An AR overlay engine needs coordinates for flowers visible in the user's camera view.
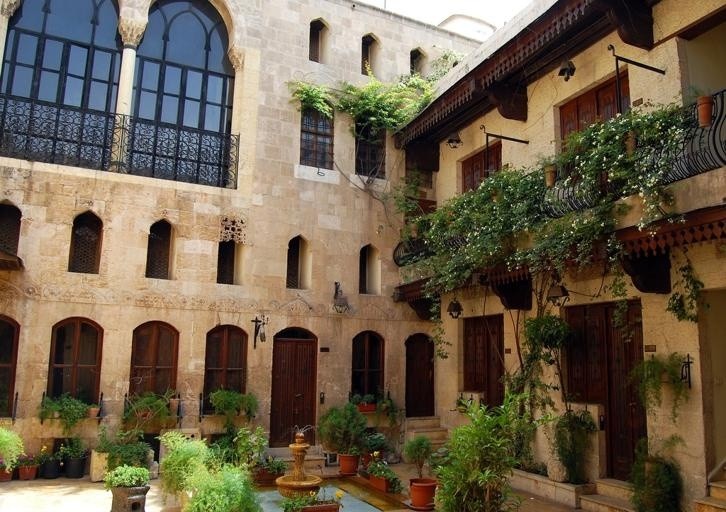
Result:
[276,489,344,512]
[380,87,710,358]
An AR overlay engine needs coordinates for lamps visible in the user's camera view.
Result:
[333,282,351,314]
[250,314,270,350]
[558,52,576,81]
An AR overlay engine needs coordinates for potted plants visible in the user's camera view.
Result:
[625,431,689,512]
[317,393,466,512]
[686,84,714,128]
[0,388,286,511]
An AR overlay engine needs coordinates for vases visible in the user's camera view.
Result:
[292,500,340,512]
